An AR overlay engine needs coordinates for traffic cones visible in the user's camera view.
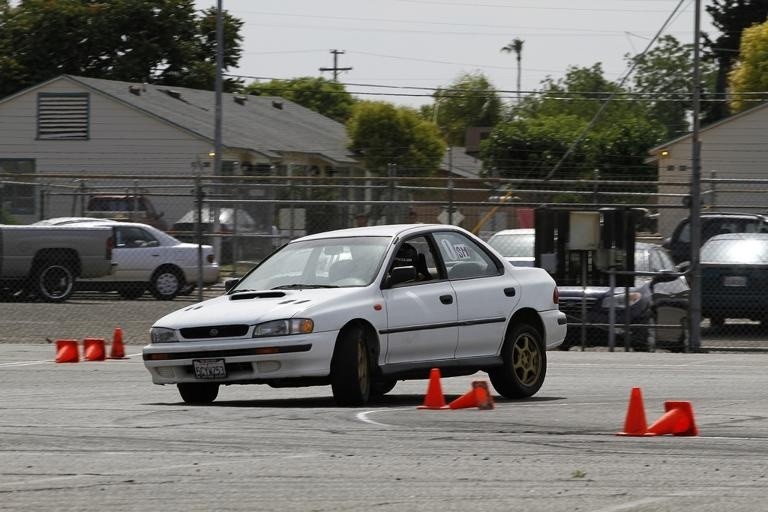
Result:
[614,386,701,441]
[51,322,129,366]
[420,361,497,413]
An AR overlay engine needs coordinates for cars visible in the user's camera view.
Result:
[0,192,261,300]
[487,213,768,350]
[141,220,571,402]
